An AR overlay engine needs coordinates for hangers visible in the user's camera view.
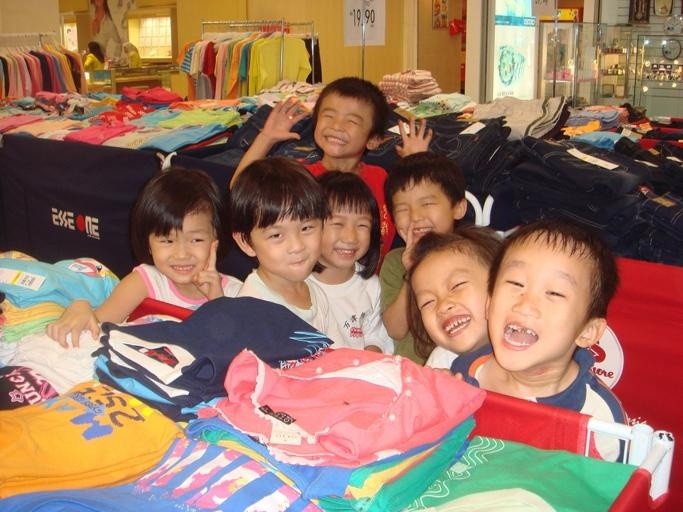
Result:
[184,20,318,47]
[229,21,315,85]
[200,19,285,82]
[0,32,81,64]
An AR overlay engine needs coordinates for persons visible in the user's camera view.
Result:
[306,171,395,355]
[230,158,348,350]
[229,77,433,276]
[379,151,468,367]
[429,216,630,465]
[81,41,104,83]
[45,166,244,350]
[402,226,502,371]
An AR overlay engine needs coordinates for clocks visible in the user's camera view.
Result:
[661,38,681,61]
[663,15,683,35]
[653,2,672,18]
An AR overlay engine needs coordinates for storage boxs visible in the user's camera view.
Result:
[126,297,681,512]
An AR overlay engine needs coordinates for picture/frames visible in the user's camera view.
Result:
[628,0,650,25]
